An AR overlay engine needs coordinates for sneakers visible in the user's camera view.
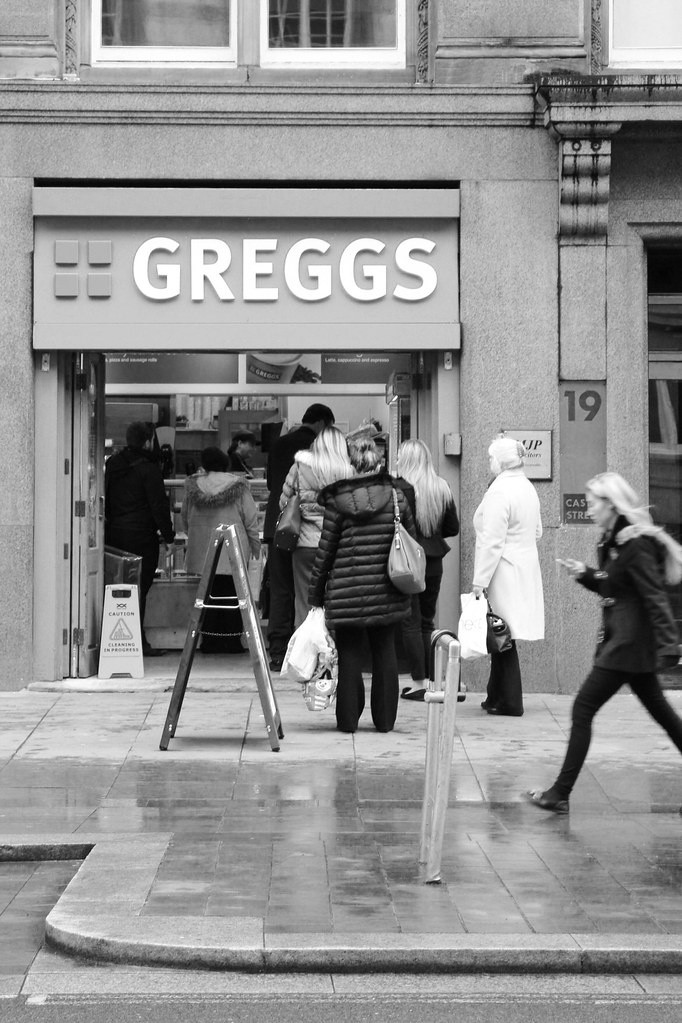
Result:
[267,659,283,671]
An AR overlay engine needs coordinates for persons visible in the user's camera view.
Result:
[224,426,258,481]
[104,418,176,658]
[306,433,418,736]
[180,445,264,655]
[471,434,549,719]
[277,426,354,640]
[516,470,681,814]
[259,399,341,675]
[385,436,466,701]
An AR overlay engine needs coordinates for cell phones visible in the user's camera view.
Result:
[556,559,574,569]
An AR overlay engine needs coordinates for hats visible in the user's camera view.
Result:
[234,430,262,445]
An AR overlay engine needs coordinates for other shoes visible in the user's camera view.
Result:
[340,727,357,732]
[376,724,395,732]
[486,707,503,714]
[481,701,495,710]
[400,686,427,701]
[522,789,570,815]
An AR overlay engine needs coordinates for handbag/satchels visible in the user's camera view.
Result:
[302,627,339,710]
[273,494,302,552]
[279,607,322,681]
[387,522,427,593]
[458,592,488,661]
[487,613,513,653]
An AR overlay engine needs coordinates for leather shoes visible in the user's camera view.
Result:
[141,643,166,657]
